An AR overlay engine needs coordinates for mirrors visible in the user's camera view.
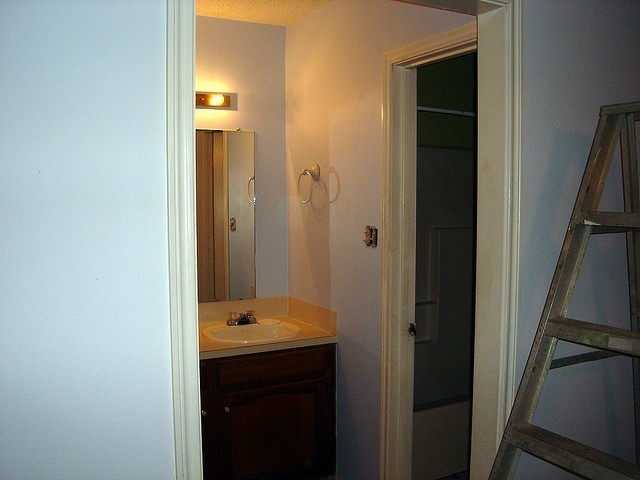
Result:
[197,130,257,304]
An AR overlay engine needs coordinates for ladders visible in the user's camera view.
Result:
[489,101,639,476]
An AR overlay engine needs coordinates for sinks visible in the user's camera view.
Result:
[202,318,303,343]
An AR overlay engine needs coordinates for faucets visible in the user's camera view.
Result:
[238,312,252,325]
